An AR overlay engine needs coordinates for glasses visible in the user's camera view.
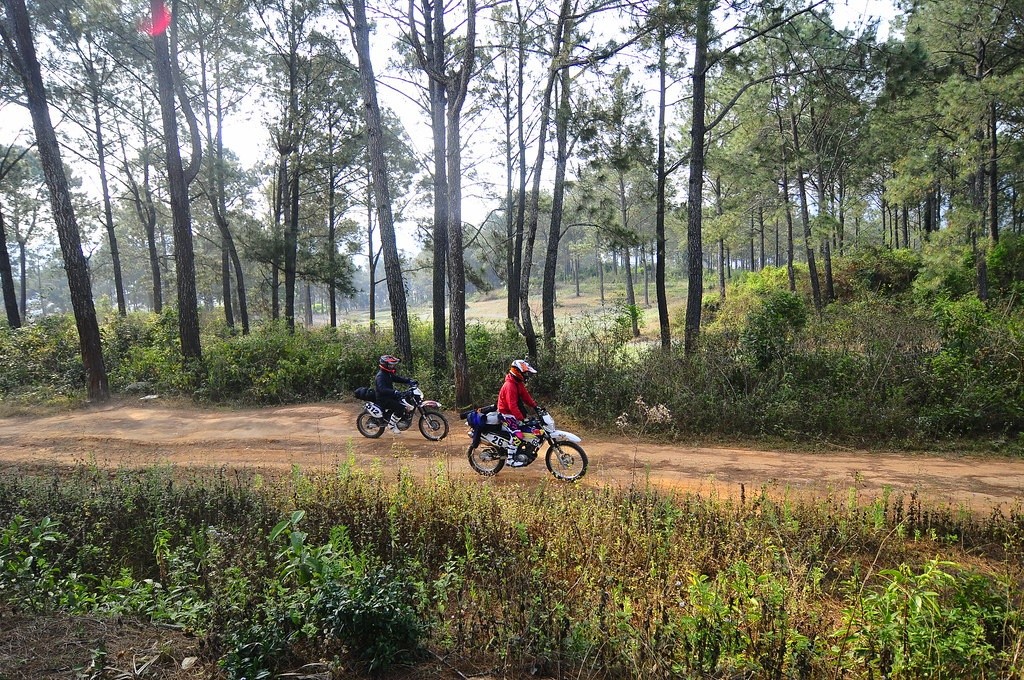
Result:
[523,372,532,377]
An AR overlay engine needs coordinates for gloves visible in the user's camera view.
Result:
[394,390,402,397]
[409,380,418,387]
[534,406,542,413]
[522,418,530,425]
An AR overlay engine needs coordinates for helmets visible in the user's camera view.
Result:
[379,355,400,374]
[509,360,537,382]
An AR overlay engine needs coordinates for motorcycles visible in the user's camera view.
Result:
[464,402,588,482]
[353,382,449,441]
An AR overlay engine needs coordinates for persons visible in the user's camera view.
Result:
[496,360,539,467]
[374,354,415,435]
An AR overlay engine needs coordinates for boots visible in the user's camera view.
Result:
[387,412,401,434]
[506,445,524,467]
